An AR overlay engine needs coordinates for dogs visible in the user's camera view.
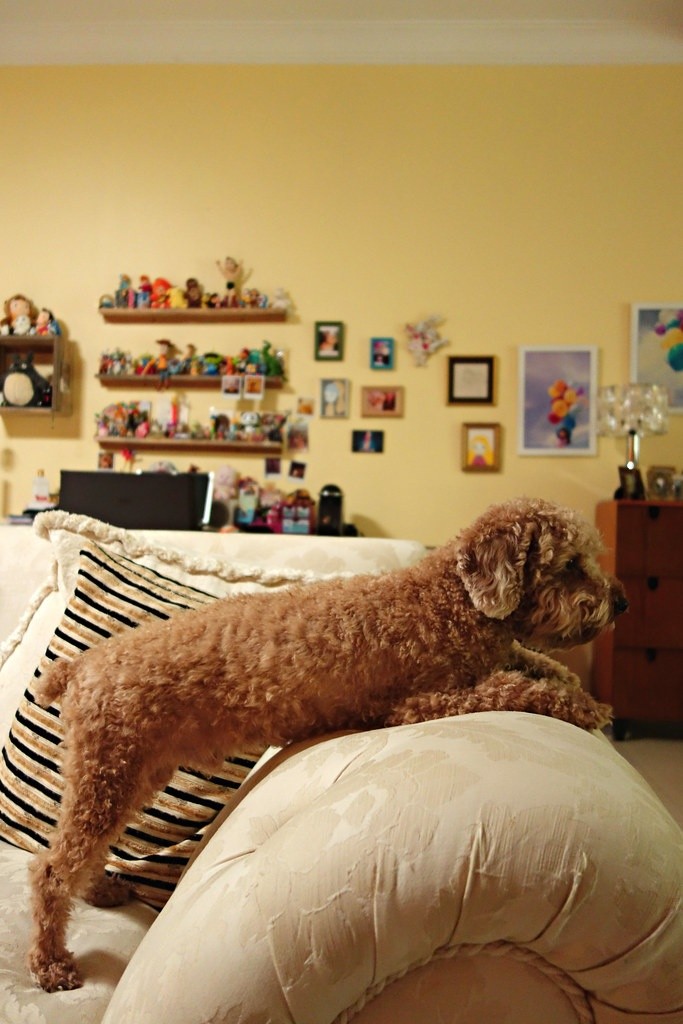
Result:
[30,495,628,984]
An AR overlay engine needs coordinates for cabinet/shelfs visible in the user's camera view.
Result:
[589,500,683,742]
[96,305,288,455]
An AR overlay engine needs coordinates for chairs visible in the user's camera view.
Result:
[22,469,217,534]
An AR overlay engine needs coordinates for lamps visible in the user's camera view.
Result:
[593,382,670,469]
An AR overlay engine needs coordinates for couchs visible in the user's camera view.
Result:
[1,521,683,1024]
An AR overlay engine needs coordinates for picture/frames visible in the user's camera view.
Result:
[628,300,682,416]
[448,355,494,405]
[516,345,599,457]
[314,320,344,362]
[461,422,501,473]
[320,377,349,419]
[369,336,395,371]
[361,386,405,418]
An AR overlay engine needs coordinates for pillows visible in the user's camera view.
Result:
[1,509,351,915]
